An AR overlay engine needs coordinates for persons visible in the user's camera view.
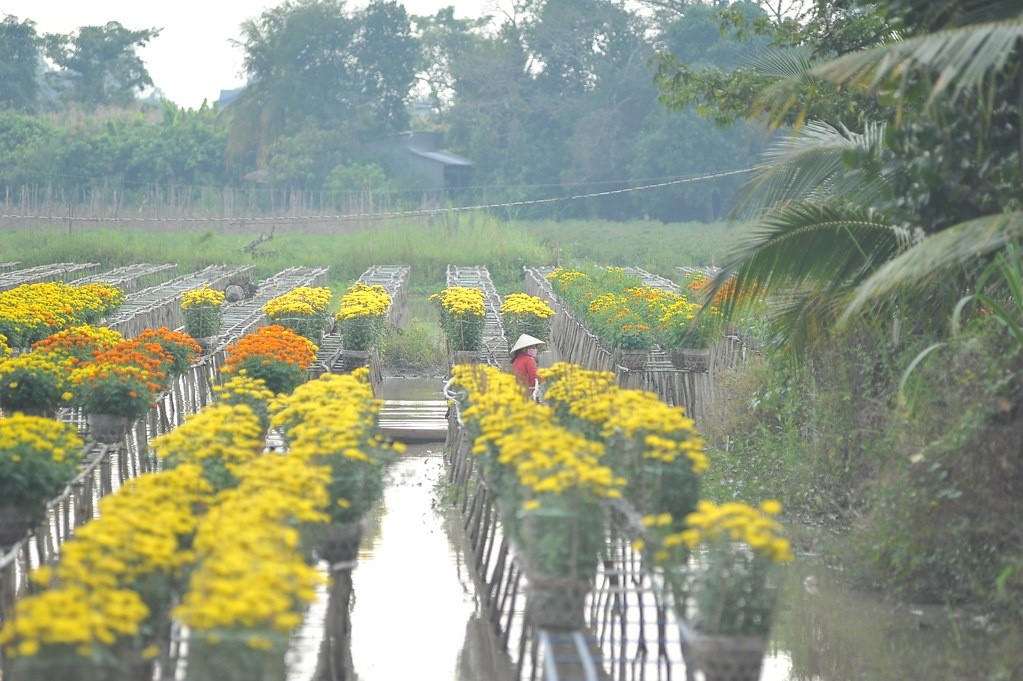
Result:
[509,334,546,400]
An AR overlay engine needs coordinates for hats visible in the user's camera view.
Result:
[510,334,546,354]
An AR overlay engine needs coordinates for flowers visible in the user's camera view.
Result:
[0,268,792,681]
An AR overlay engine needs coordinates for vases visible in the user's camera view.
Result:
[684,348,712,373]
[225,285,244,302]
[525,570,588,628]
[677,621,768,681]
[88,414,128,445]
[624,351,646,369]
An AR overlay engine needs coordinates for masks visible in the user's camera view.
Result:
[527,348,538,356]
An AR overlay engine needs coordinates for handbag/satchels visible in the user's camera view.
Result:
[528,378,545,403]
